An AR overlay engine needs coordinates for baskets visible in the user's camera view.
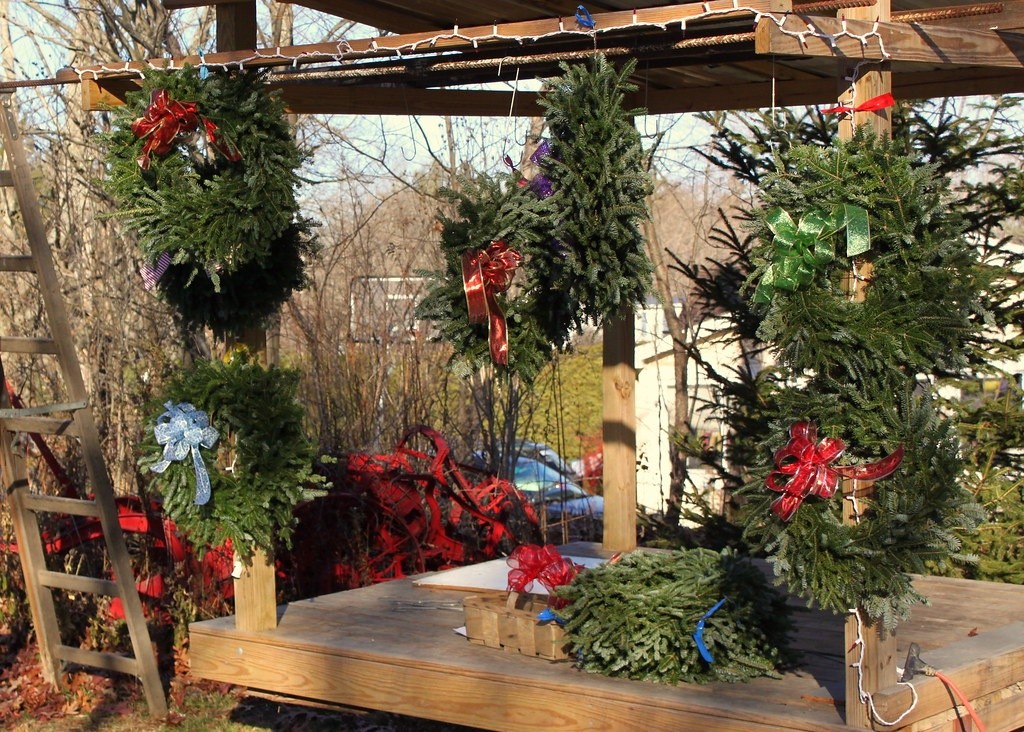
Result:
[462,554,576,660]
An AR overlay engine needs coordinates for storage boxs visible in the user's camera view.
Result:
[463,591,569,661]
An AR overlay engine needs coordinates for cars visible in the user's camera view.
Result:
[473,454,604,532]
[467,439,580,488]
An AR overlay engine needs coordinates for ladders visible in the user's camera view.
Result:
[0,88,170,722]
